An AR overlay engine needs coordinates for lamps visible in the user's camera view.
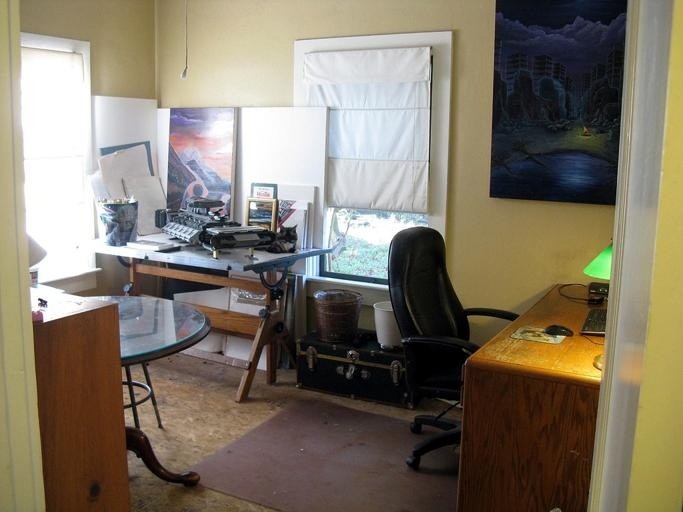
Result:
[581,237,614,281]
[26,233,48,268]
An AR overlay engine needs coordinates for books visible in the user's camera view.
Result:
[275,182,317,250]
[95,141,168,237]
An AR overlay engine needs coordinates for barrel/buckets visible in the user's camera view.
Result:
[314,289,363,343]
[97,199,138,246]
[373,301,402,346]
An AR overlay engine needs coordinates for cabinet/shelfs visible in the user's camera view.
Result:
[454,282,651,512]
[29,283,132,512]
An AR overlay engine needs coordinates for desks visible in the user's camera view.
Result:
[75,232,334,403]
[80,294,212,431]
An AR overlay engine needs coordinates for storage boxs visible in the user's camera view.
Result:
[295,328,425,411]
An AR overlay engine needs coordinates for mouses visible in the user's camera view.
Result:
[544,325,573,336]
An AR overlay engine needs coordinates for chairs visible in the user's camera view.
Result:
[387,227,521,470]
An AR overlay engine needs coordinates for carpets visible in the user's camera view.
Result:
[182,397,461,512]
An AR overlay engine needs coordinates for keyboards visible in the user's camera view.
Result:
[579,308,607,336]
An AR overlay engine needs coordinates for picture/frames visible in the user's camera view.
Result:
[245,182,278,232]
